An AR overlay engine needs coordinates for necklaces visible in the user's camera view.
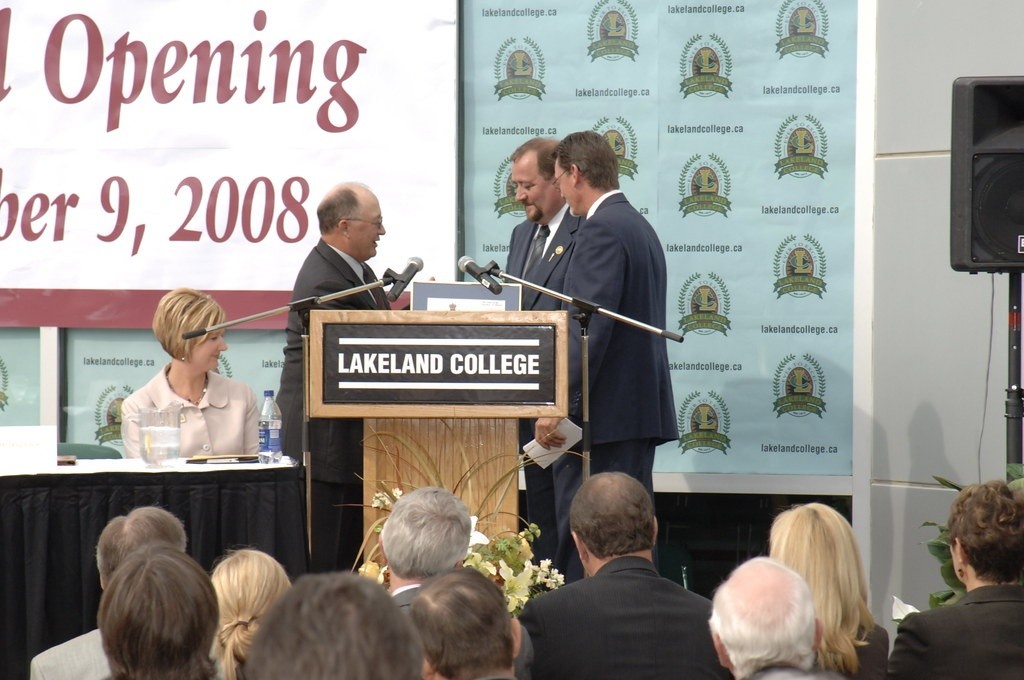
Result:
[166,373,208,405]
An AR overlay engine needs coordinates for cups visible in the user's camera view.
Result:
[136,408,182,465]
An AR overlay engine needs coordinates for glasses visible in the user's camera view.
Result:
[341,217,383,228]
[552,167,579,189]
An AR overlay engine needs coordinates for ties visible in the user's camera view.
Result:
[361,264,387,310]
[524,224,551,281]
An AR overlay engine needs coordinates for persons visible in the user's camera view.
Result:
[211,549,292,680]
[380,486,534,680]
[518,472,735,680]
[409,567,522,680]
[888,480,1024,680]
[275,183,392,571]
[29,506,186,680]
[243,572,423,680]
[709,556,824,680]
[507,139,585,567]
[536,130,679,590]
[120,289,260,459]
[769,503,890,680]
[97,541,221,680]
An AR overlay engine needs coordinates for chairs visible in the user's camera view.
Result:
[58,443,123,459]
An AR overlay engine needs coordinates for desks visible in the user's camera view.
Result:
[0,454,311,680]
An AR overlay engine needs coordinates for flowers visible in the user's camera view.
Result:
[331,419,593,616]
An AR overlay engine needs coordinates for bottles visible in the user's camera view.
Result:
[257,389,282,465]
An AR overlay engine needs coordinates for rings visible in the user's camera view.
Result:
[546,434,550,436]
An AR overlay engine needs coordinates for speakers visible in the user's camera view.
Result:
[951,75,1024,275]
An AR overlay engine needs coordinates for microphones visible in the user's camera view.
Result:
[386,257,424,302]
[457,256,502,295]
